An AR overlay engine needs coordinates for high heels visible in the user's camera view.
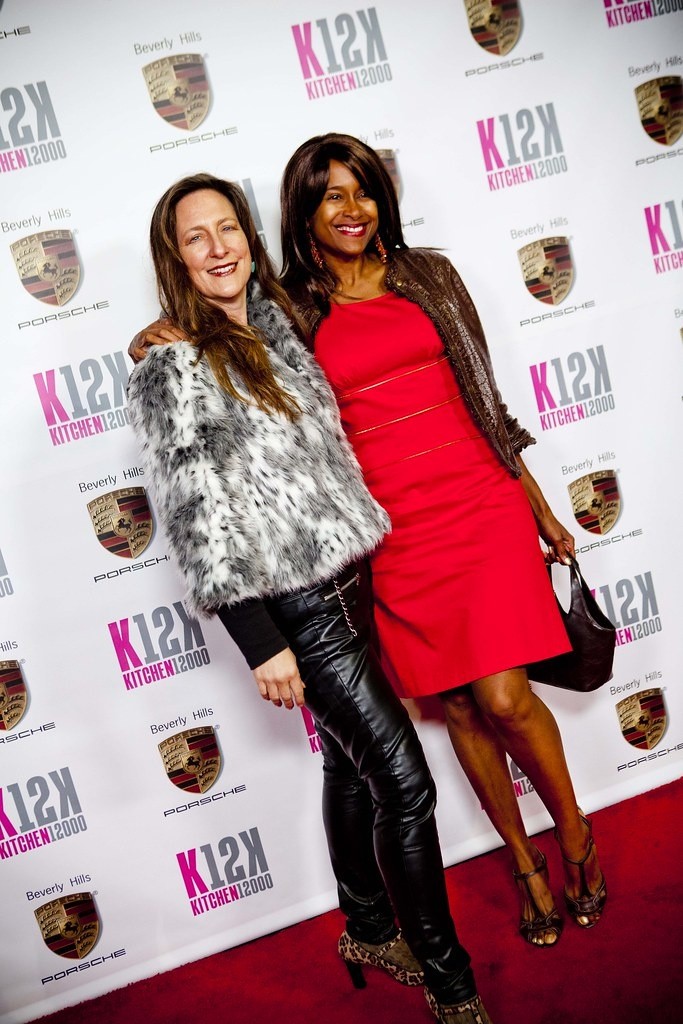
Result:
[554,814,606,928]
[512,845,563,945]
[337,927,424,990]
[424,985,491,1024]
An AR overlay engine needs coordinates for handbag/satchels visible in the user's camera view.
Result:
[526,551,617,692]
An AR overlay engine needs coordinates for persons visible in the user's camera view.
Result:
[127,133,608,947]
[125,172,490,1024]
